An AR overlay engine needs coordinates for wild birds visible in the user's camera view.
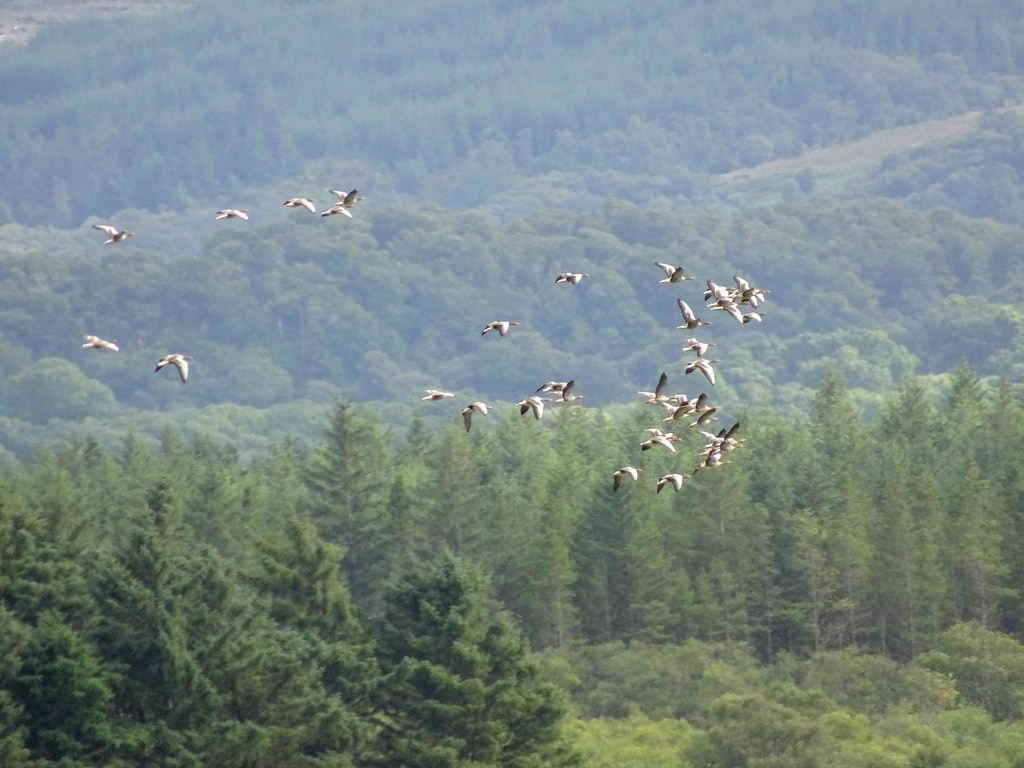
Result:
[214,208,249,222]
[535,381,567,394]
[329,190,366,207]
[636,272,771,475]
[652,260,695,285]
[320,204,353,219]
[481,321,519,336]
[555,380,584,404]
[462,401,493,434]
[422,389,455,401]
[283,197,317,213]
[610,466,642,491]
[154,352,193,382]
[81,334,121,353]
[515,395,552,421]
[555,272,589,284]
[655,472,691,494]
[91,224,133,246]
